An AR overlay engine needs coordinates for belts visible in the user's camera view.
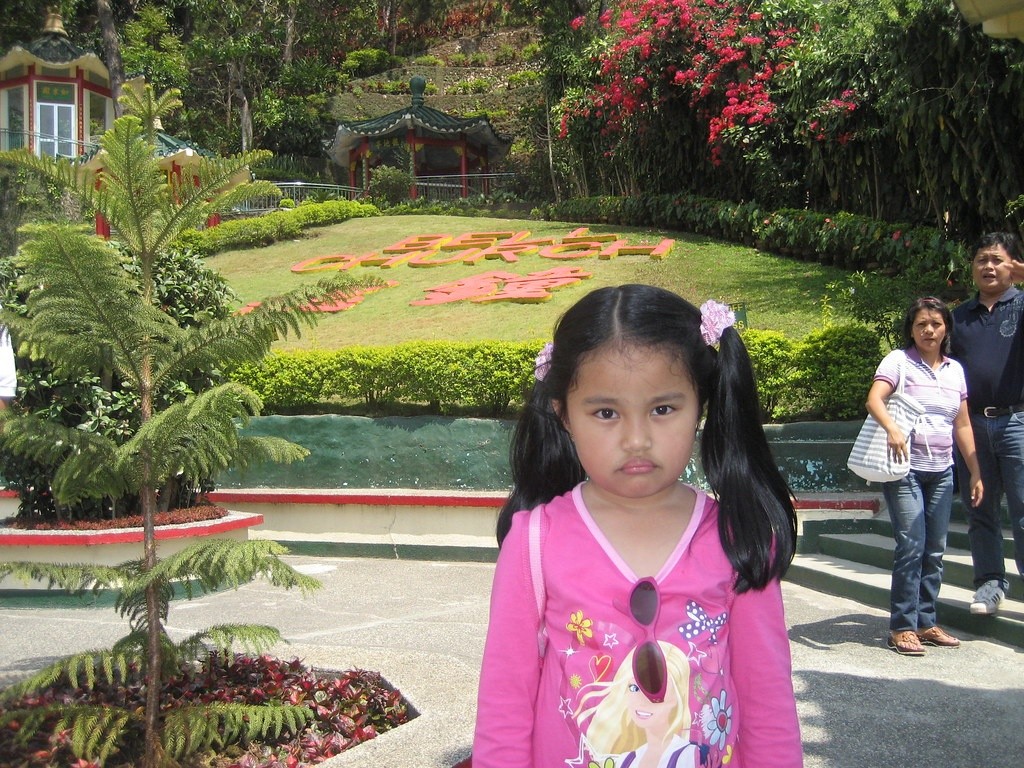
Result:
[969,403,1022,419]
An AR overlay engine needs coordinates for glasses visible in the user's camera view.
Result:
[630,577,668,703]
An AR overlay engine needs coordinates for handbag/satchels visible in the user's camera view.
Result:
[846,349,927,483]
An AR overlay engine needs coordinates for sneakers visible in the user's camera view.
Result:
[916,626,959,648]
[970,577,1009,615]
[887,625,927,655]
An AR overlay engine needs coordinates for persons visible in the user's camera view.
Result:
[471,285,804,768]
[942,232,1024,616]
[864,295,984,656]
